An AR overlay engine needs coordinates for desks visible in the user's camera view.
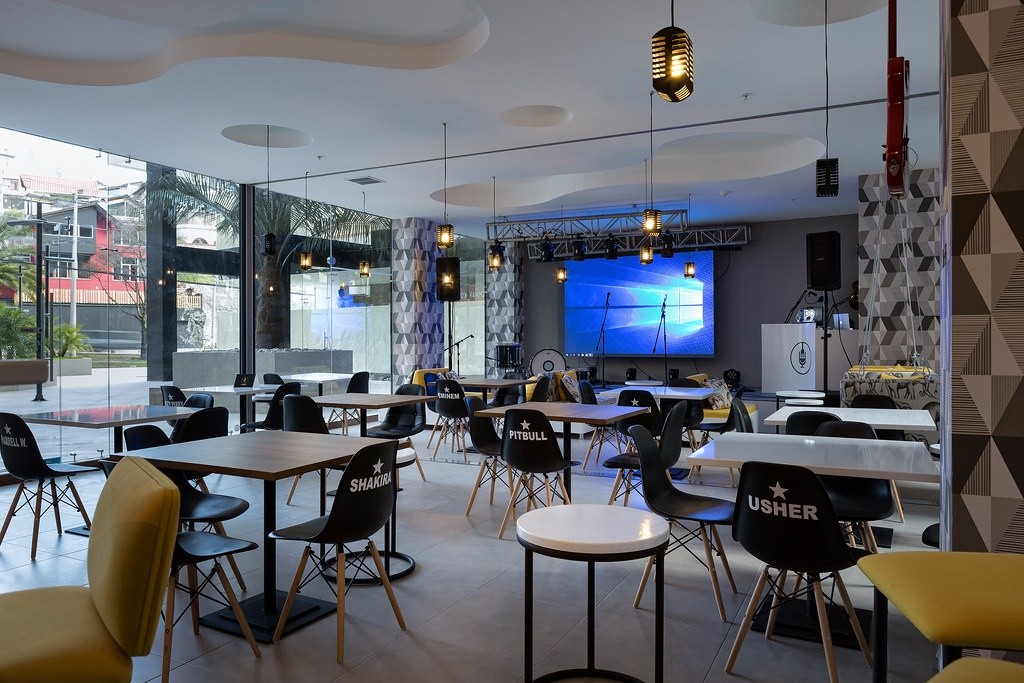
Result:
[21,372,941,682]
[761,323,859,393]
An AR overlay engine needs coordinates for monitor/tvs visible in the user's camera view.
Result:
[800,307,824,329]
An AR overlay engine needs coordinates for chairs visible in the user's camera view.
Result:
[0,373,895,683]
[855,550,1024,683]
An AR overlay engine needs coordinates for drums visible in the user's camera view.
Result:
[529,347,566,376]
[576,370,589,381]
[494,342,525,368]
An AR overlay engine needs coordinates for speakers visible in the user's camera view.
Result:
[436,256,460,302]
[805,231,841,291]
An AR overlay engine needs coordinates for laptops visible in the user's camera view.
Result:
[833,313,850,330]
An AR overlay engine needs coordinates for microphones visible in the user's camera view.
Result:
[605,292,610,305]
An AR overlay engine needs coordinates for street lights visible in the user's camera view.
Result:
[70,188,84,358]
[16,265,23,309]
[7,202,47,402]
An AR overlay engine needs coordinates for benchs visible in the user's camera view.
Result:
[378,369,618,440]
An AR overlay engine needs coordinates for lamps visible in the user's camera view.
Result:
[571,235,585,261]
[489,177,501,268]
[684,194,695,279]
[301,172,312,270]
[642,91,662,237]
[436,123,454,249]
[604,233,617,259]
[640,238,653,265]
[555,205,567,283]
[360,192,369,278]
[661,230,673,258]
[443,249,452,287]
[265,125,276,256]
[815,0,839,197]
[539,232,555,262]
[723,369,741,388]
[652,0,695,103]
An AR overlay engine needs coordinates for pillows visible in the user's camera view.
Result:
[436,372,446,380]
[525,375,538,401]
[561,373,581,403]
[444,370,464,392]
[556,369,578,401]
[403,371,413,384]
